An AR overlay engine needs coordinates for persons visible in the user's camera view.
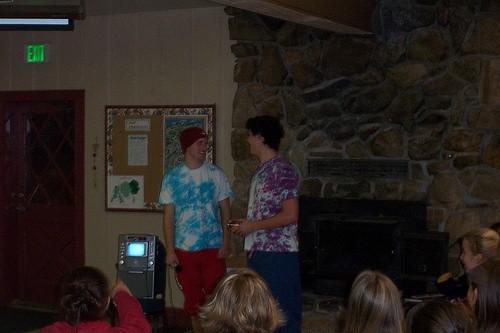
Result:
[159,127,234,318]
[190,269,283,333]
[33,266,152,333]
[339,221,500,333]
[227,114,302,333]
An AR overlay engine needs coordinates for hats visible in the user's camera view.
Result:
[179,127,208,154]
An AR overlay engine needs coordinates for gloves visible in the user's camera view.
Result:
[437,272,452,284]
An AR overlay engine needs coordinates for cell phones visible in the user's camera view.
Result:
[227,224,240,227]
